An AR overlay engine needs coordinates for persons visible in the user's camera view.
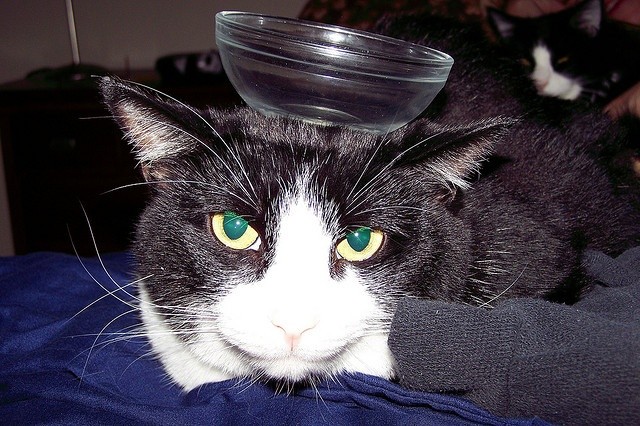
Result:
[603,83,640,177]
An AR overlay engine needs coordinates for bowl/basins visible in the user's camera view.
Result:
[214,11,454,135]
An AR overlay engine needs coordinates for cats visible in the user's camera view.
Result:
[98,2,640,395]
[483,0,640,118]
